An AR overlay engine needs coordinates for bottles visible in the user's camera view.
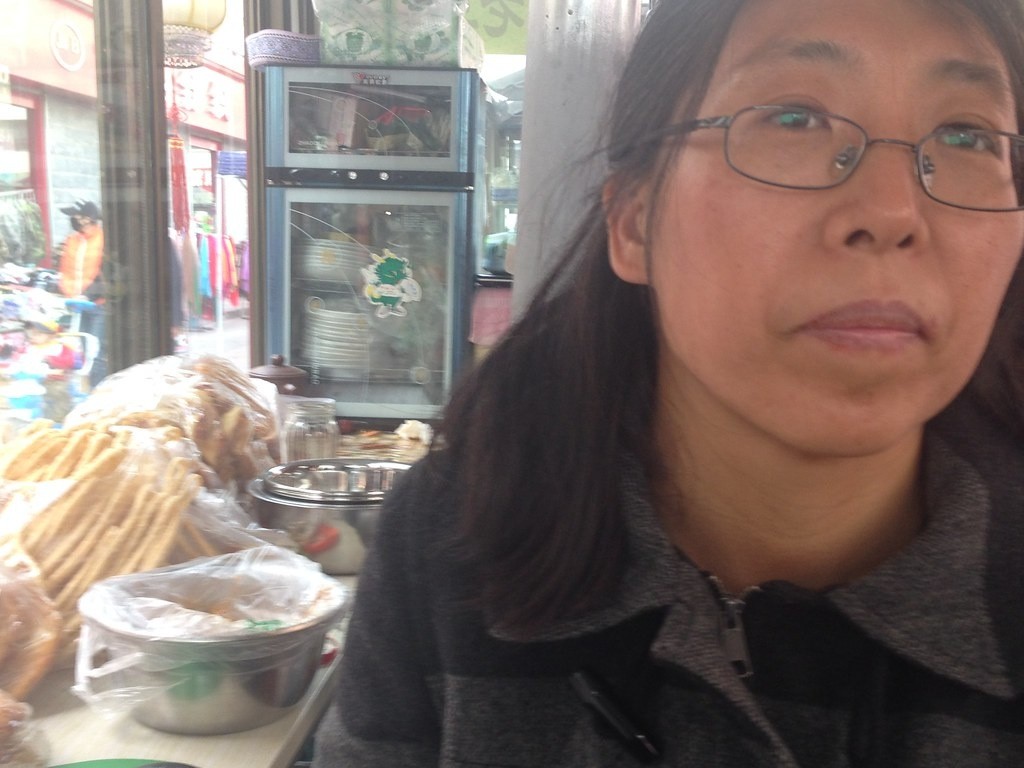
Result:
[280,396,339,461]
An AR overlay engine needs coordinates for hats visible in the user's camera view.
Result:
[60,198,98,219]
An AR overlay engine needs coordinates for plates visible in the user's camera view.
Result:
[290,237,377,379]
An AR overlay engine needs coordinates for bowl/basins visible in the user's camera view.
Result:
[76,575,348,736]
[245,456,412,577]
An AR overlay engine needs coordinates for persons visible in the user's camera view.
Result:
[310,1,1024,766]
[79,236,182,355]
[55,198,107,389]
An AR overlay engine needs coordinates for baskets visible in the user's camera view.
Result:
[247,29,323,71]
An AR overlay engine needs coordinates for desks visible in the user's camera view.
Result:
[26,655,344,768]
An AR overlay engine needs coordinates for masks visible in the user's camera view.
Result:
[70,217,93,231]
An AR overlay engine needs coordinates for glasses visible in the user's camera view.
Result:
[658,104,1024,212]
